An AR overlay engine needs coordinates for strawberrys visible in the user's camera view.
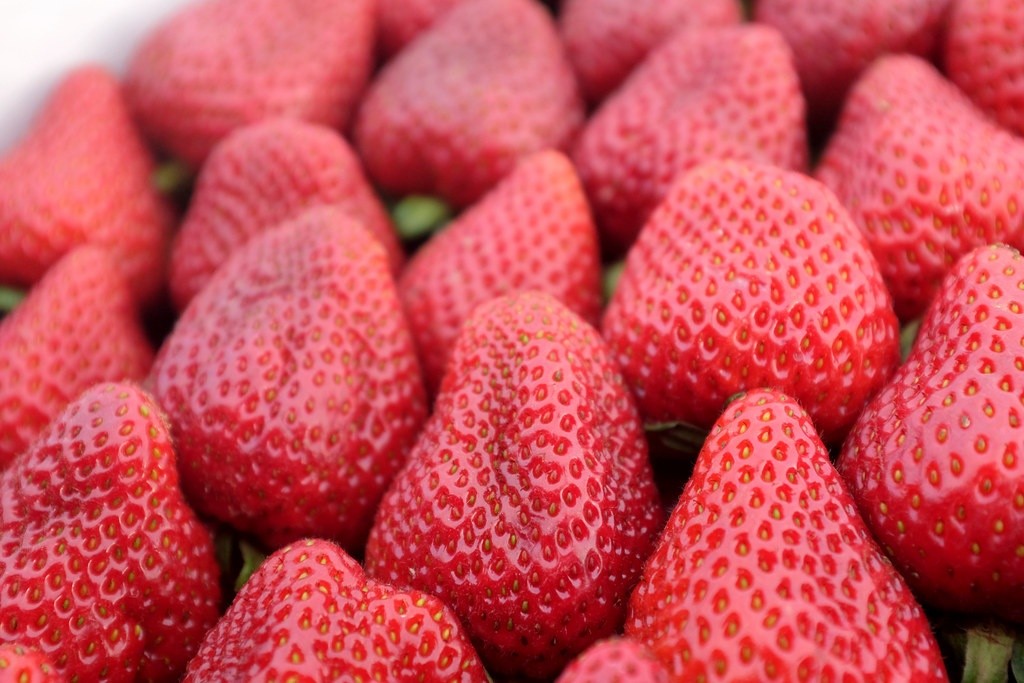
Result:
[0,0,1024,683]
